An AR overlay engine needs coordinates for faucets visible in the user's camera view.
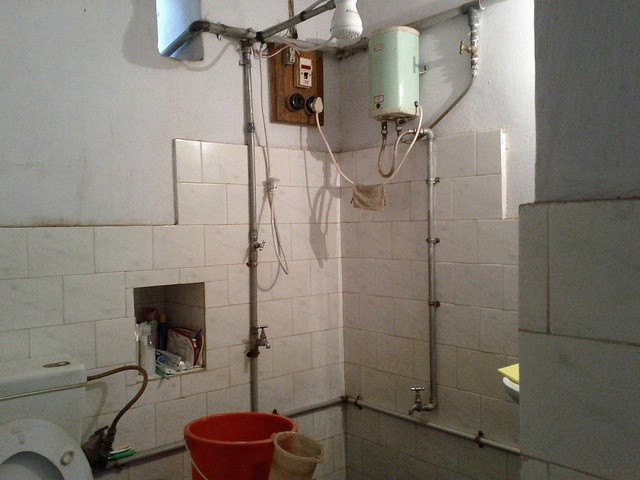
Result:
[255,325,271,350]
[408,387,424,414]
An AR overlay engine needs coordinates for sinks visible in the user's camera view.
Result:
[0,356,97,480]
[501,375,520,405]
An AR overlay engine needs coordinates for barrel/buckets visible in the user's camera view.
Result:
[183,412,298,480]
